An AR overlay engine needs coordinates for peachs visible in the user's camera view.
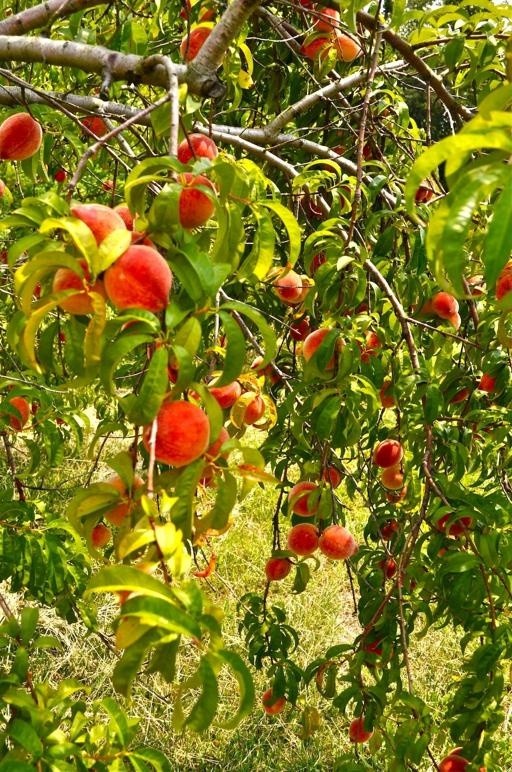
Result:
[1,1,511,772]
